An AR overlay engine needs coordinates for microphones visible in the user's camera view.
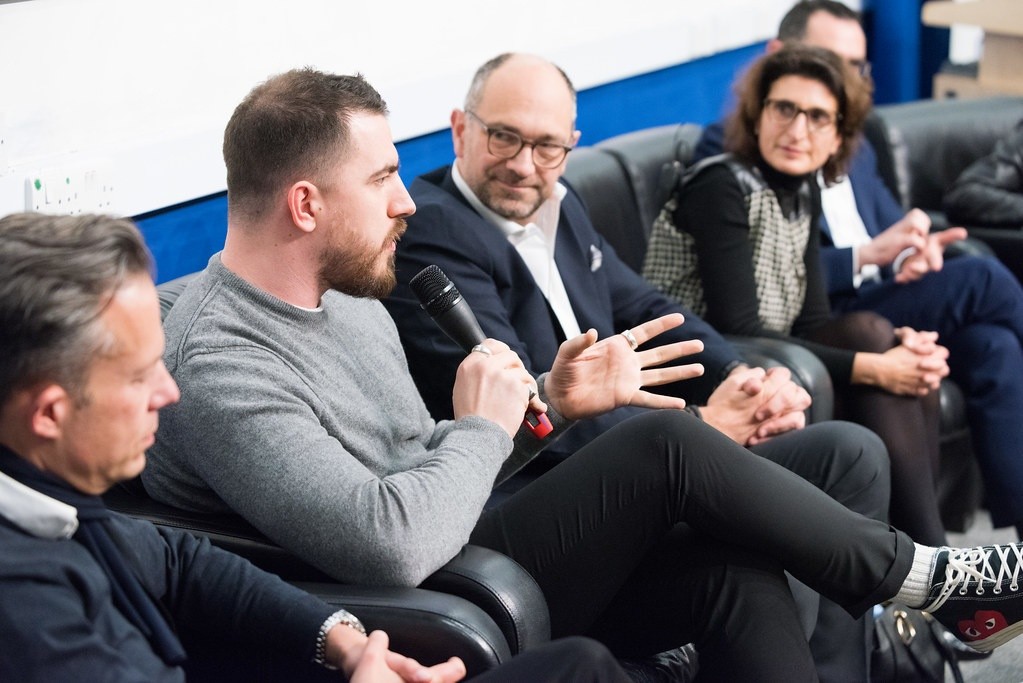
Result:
[409,264,554,439]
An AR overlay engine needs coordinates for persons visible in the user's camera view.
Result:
[379,52,891,682]
[638,38,996,659]
[141,68,1023,682]
[313,608,367,670]
[767,0,1023,541]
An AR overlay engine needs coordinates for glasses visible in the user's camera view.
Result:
[466,109,573,171]
[763,97,844,131]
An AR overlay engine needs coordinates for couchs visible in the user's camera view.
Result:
[0,91,1023,683]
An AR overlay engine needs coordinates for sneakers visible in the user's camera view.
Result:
[903,541,1023,652]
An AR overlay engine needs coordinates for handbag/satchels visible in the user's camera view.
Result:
[870,601,963,683]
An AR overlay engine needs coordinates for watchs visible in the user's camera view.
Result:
[0,213,633,683]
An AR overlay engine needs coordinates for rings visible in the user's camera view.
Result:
[621,329,638,350]
